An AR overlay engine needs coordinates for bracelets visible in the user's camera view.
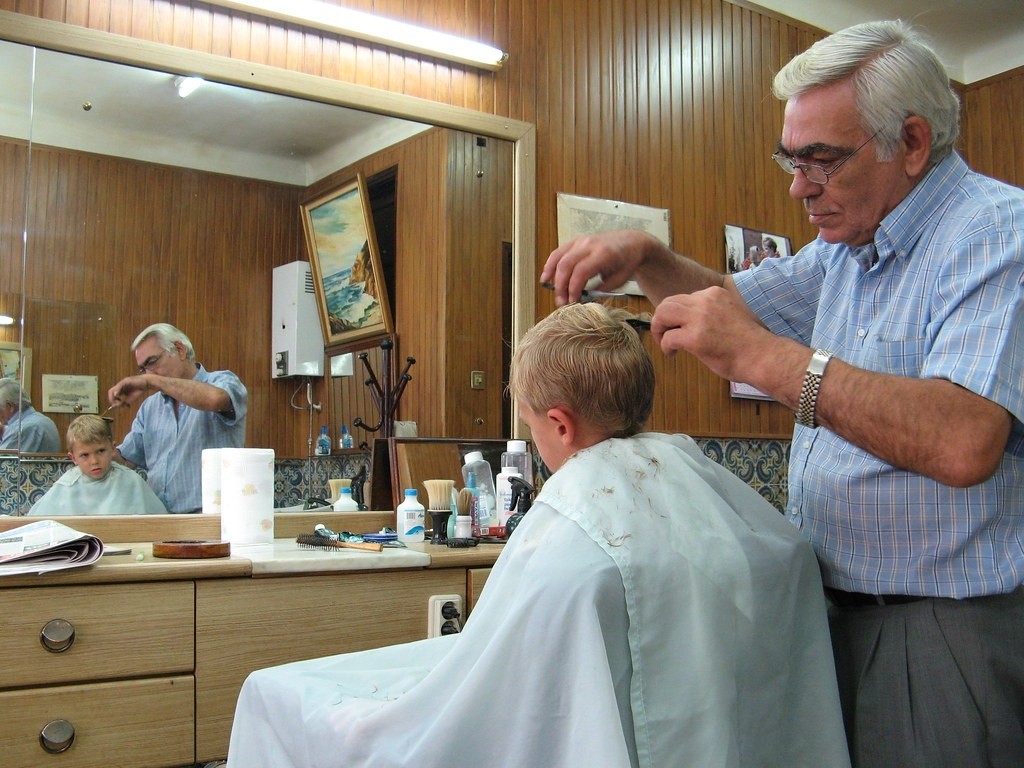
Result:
[792,348,830,428]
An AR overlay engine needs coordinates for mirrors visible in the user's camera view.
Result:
[0,9,538,543]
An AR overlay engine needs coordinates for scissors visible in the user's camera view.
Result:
[539,278,632,302]
[102,392,131,416]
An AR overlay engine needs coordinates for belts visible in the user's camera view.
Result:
[823,584,930,608]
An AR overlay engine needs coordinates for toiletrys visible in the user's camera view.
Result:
[315,424,331,455]
[339,423,353,449]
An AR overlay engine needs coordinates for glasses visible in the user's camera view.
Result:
[137,349,167,373]
[771,124,886,185]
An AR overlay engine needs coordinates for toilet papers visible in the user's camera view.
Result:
[221,448,275,541]
[201,447,220,515]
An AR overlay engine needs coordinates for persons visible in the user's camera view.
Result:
[109,323,248,515]
[540,20,1023,767]
[226,302,851,768]
[27,415,168,515]
[741,237,781,269]
[0,378,61,452]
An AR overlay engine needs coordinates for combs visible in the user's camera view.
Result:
[628,317,652,330]
[297,535,383,552]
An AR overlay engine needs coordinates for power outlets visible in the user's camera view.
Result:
[427,594,462,636]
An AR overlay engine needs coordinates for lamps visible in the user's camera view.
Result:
[197,0,509,72]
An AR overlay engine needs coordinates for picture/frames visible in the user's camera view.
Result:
[298,170,394,357]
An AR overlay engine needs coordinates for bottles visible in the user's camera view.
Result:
[338,424,352,447]
[396,489,425,543]
[461,472,481,537]
[455,515,472,538]
[332,488,358,513]
[497,467,524,528]
[501,441,535,506]
[314,426,330,456]
[460,450,500,536]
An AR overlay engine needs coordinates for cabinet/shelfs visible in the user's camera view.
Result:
[1,530,507,768]
